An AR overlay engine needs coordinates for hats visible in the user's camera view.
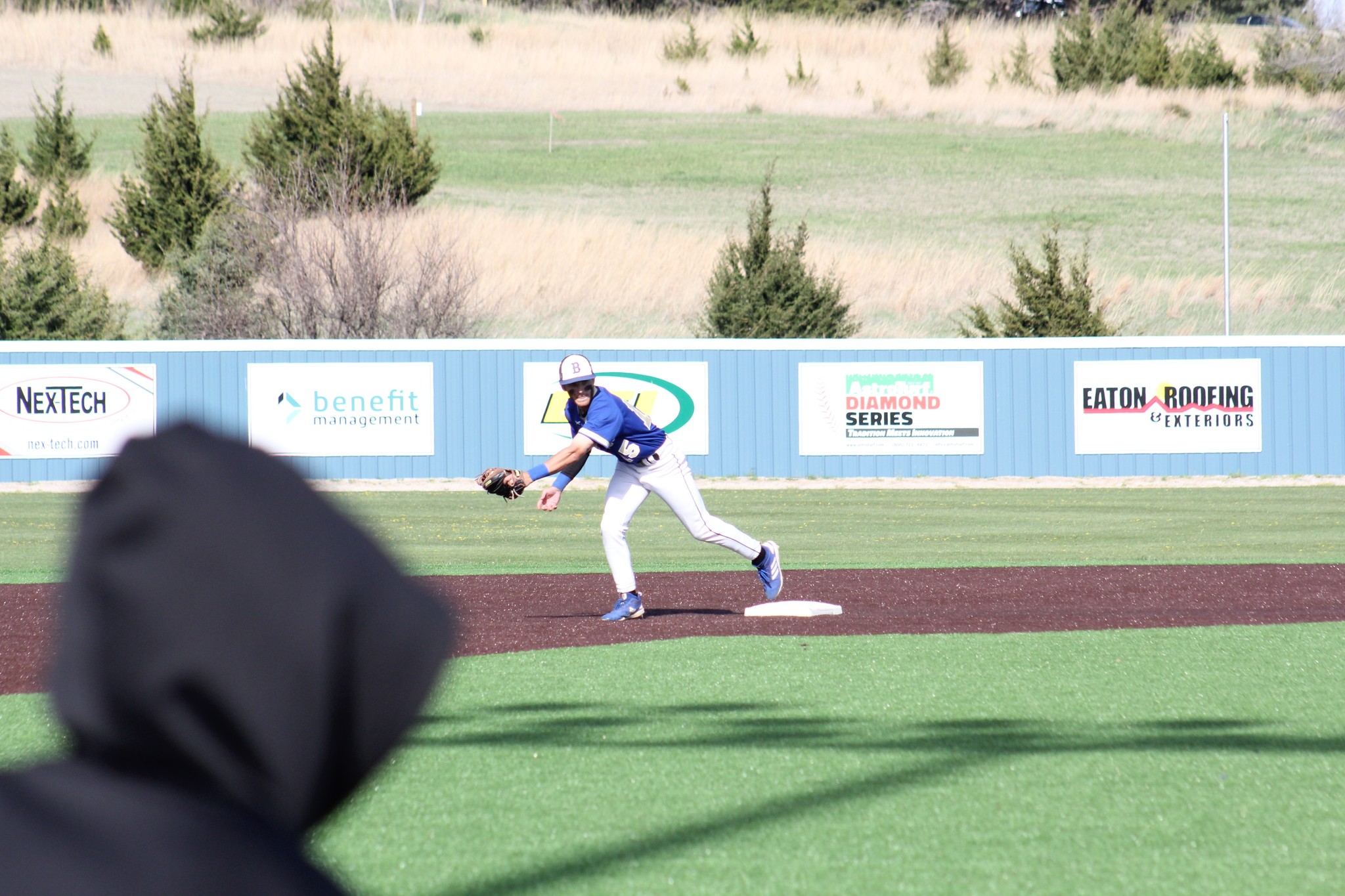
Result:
[559,354,596,385]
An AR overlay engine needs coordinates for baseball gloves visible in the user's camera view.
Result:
[476,468,526,500]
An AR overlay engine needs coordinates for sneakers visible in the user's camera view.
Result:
[751,540,783,600]
[601,593,645,621]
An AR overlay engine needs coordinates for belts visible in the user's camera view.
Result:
[634,453,660,467]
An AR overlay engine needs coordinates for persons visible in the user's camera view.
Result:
[502,354,783,620]
[0,425,455,896]
